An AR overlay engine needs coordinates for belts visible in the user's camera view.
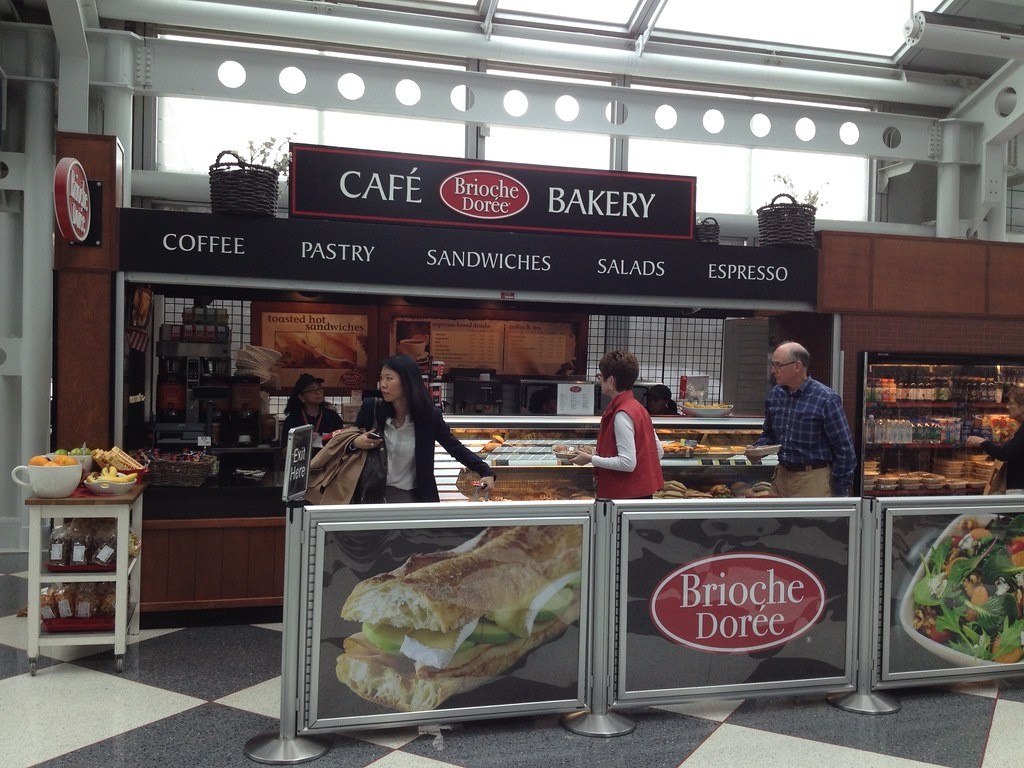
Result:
[779,463,831,472]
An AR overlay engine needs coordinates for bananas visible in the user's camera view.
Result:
[87,466,138,486]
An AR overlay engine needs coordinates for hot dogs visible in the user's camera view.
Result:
[336,524,583,711]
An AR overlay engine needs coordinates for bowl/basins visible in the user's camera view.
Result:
[677,402,734,417]
[745,443,781,456]
[399,338,425,357]
[864,452,994,489]
[234,344,283,384]
[117,468,146,484]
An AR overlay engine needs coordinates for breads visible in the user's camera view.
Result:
[684,402,729,408]
[653,480,771,499]
[485,435,505,451]
[663,442,709,452]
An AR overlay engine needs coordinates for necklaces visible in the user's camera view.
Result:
[395,420,401,427]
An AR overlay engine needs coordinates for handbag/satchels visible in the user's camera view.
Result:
[352,397,387,499]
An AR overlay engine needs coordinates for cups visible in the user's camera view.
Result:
[11,464,83,498]
[39,453,93,480]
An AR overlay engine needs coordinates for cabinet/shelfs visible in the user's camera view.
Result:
[854,350,1024,496]
[24,484,149,676]
[434,414,781,501]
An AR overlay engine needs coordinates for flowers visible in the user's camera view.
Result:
[773,172,830,208]
[696,217,702,225]
[247,132,297,196]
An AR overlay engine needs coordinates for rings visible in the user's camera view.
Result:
[371,442,374,447]
[374,444,377,447]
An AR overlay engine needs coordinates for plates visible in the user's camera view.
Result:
[83,473,138,495]
[898,514,1024,668]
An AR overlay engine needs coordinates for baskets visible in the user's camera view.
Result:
[147,453,217,487]
[756,194,817,247]
[696,217,721,245]
[209,150,280,217]
[119,468,146,484]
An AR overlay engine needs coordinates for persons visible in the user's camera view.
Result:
[742,341,858,497]
[280,373,344,459]
[528,387,557,415]
[322,353,497,504]
[644,384,681,415]
[567,348,664,499]
[966,384,1024,495]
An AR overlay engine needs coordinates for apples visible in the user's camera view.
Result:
[55,447,91,455]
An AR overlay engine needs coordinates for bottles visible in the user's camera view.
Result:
[211,404,221,417]
[240,403,252,418]
[162,403,178,417]
[866,376,1017,445]
[211,423,220,444]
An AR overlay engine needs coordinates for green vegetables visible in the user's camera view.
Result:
[915,512,1024,659]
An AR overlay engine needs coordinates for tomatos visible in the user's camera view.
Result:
[949,536,962,545]
[1011,541,1024,553]
[931,624,952,643]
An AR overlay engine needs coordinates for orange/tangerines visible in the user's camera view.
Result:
[29,455,78,467]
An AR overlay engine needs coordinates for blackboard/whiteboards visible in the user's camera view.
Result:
[281,424,313,502]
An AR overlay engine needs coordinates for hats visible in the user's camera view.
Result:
[296,373,325,384]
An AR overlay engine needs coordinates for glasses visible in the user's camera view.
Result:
[301,386,326,395]
[769,360,798,370]
[596,372,609,380]
